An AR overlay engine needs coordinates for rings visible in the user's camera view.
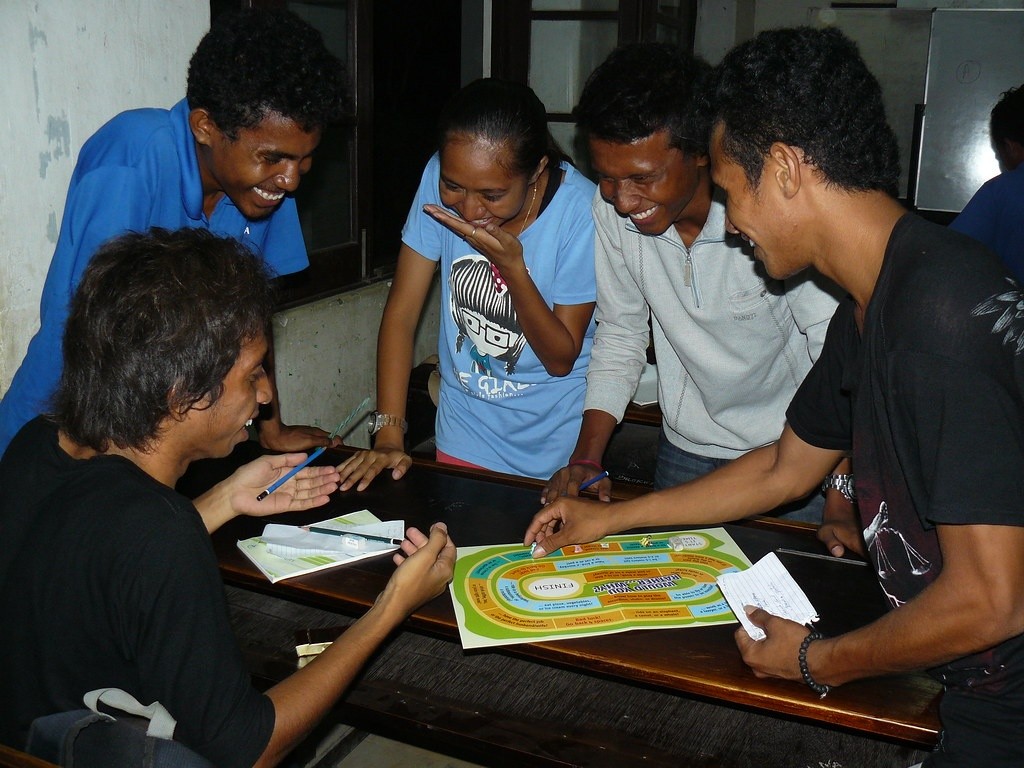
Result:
[463,234,466,240]
[472,227,477,237]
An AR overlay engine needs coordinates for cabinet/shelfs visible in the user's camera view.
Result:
[490,0,703,123]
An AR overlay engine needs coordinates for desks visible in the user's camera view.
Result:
[403,360,663,463]
[175,440,944,768]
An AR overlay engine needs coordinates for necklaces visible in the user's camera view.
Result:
[518,181,537,233]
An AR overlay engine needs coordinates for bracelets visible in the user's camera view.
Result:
[570,459,603,470]
[798,631,833,693]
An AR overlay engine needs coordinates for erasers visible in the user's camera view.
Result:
[342,534,366,548]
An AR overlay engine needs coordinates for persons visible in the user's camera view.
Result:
[524,26,1024,768]
[1,0,344,462]
[0,227,457,768]
[950,85,1024,283]
[540,45,869,681]
[334,78,597,492]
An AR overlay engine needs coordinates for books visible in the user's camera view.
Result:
[237,510,406,584]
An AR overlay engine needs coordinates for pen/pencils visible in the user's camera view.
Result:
[256,445,329,502]
[560,470,610,506]
[297,525,403,545]
[308,397,370,456]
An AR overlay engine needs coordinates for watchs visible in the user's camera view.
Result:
[821,474,857,506]
[368,410,408,434]
